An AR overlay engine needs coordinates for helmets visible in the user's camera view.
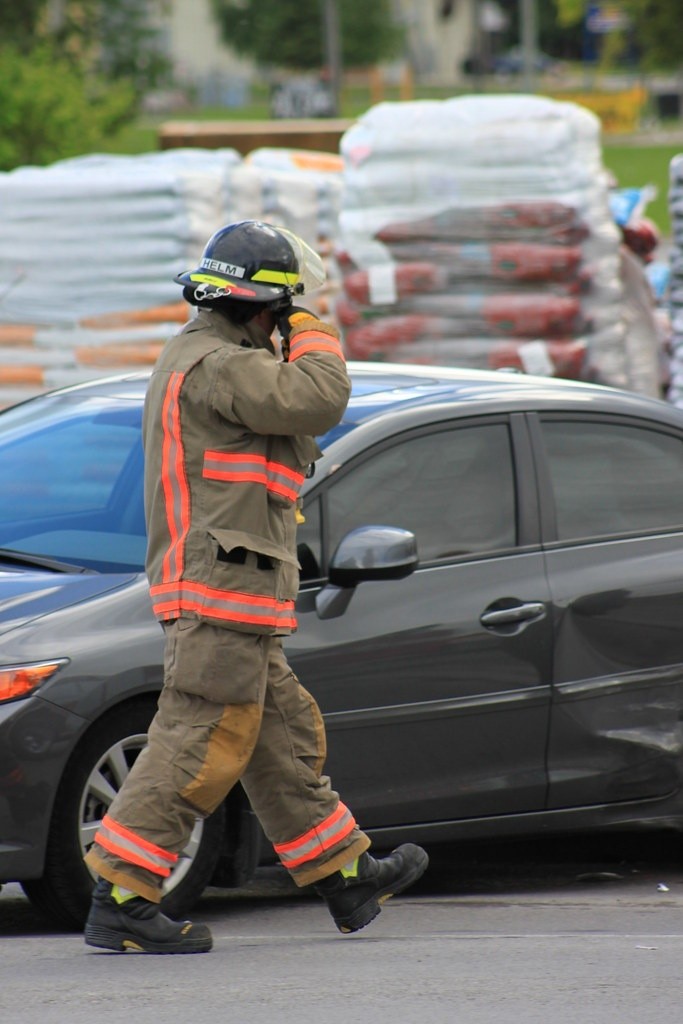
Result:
[173,220,305,301]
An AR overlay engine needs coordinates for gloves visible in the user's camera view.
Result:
[276,305,321,363]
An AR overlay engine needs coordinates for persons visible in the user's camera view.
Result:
[618,221,670,308]
[83,219,432,956]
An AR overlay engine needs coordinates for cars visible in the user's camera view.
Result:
[0,360,683,927]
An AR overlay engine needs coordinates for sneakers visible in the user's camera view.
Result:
[314,843,429,934]
[84,881,213,952]
